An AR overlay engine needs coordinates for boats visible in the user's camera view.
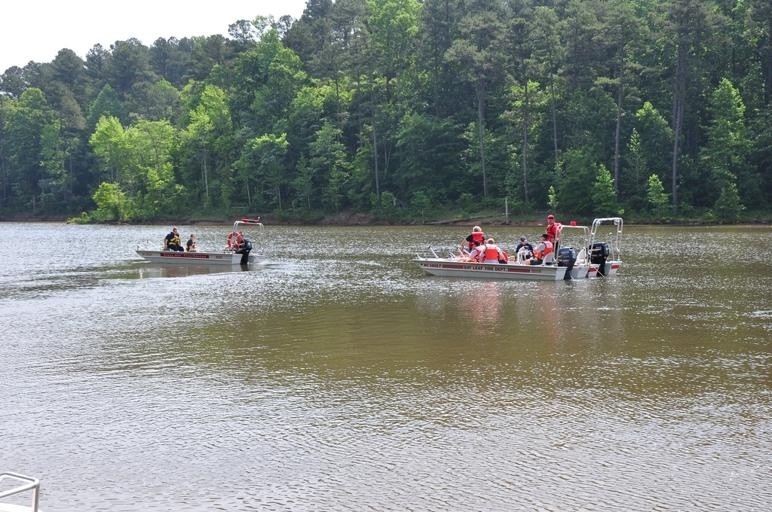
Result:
[414,222,592,281]
[0,471,41,512]
[137,217,266,265]
[445,217,623,276]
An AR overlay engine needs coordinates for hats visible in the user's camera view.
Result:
[540,234,550,238]
[546,215,555,219]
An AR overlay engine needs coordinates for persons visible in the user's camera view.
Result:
[515,214,561,265]
[186,233,197,252]
[163,227,184,251]
[458,225,508,265]
